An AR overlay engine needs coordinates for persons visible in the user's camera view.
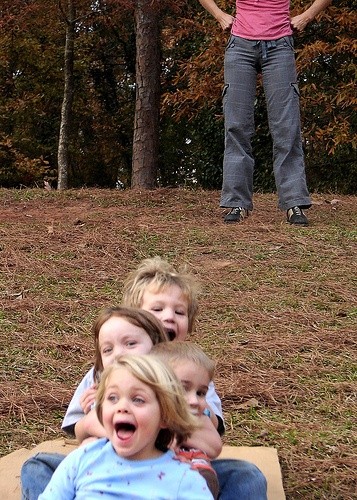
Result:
[75,340,218,500]
[196,0,331,226]
[37,352,215,500]
[19,257,268,500]
[74,305,221,461]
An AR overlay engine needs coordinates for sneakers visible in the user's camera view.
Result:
[286,206,308,225]
[225,206,249,222]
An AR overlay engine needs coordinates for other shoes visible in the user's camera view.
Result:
[192,460,219,497]
